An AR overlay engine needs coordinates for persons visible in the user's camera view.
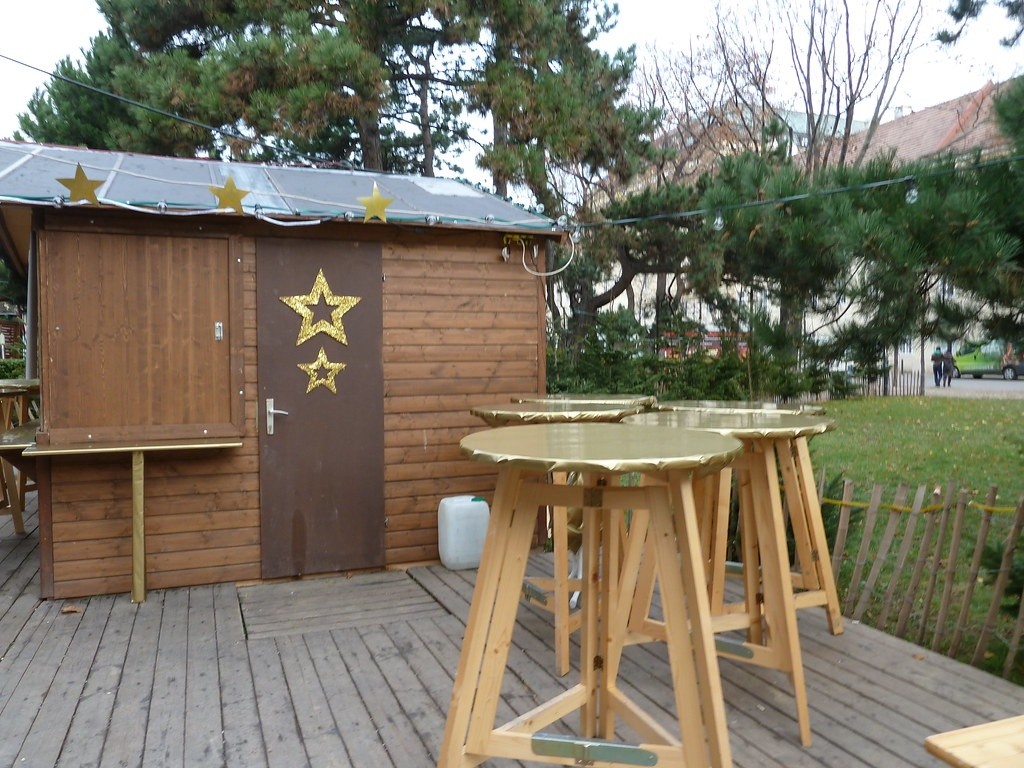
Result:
[942,350,956,388]
[931,347,944,388]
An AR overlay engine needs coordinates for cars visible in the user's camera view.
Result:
[951,338,1024,381]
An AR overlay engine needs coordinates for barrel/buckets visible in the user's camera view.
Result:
[438,495,490,571]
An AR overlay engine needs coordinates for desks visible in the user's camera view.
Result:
[433,391,843,768]
[0,419,243,599]
